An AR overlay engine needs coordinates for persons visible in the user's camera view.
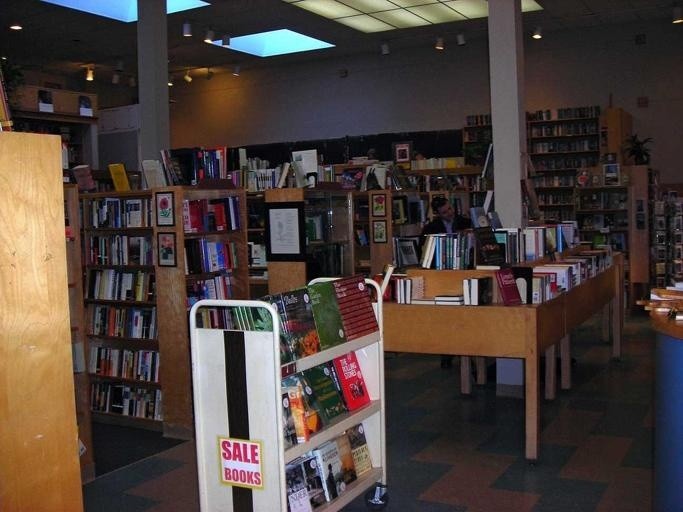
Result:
[420,197,472,260]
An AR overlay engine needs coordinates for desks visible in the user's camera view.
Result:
[370,245,624,460]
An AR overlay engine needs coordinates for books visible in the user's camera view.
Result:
[282,351,373,511]
[372,218,612,305]
[341,156,419,267]
[84,268,161,383]
[199,274,380,366]
[238,148,345,283]
[462,103,598,217]
[80,159,170,265]
[159,146,240,307]
[89,380,162,422]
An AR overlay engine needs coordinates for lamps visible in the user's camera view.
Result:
[532,26,543,40]
[434,37,445,50]
[221,33,230,45]
[203,25,215,45]
[381,43,392,56]
[672,7,683,24]
[111,73,120,85]
[232,66,241,77]
[115,60,125,73]
[85,68,95,82]
[182,18,193,37]
[206,67,214,79]
[184,70,193,83]
[455,33,466,46]
[128,75,136,89]
